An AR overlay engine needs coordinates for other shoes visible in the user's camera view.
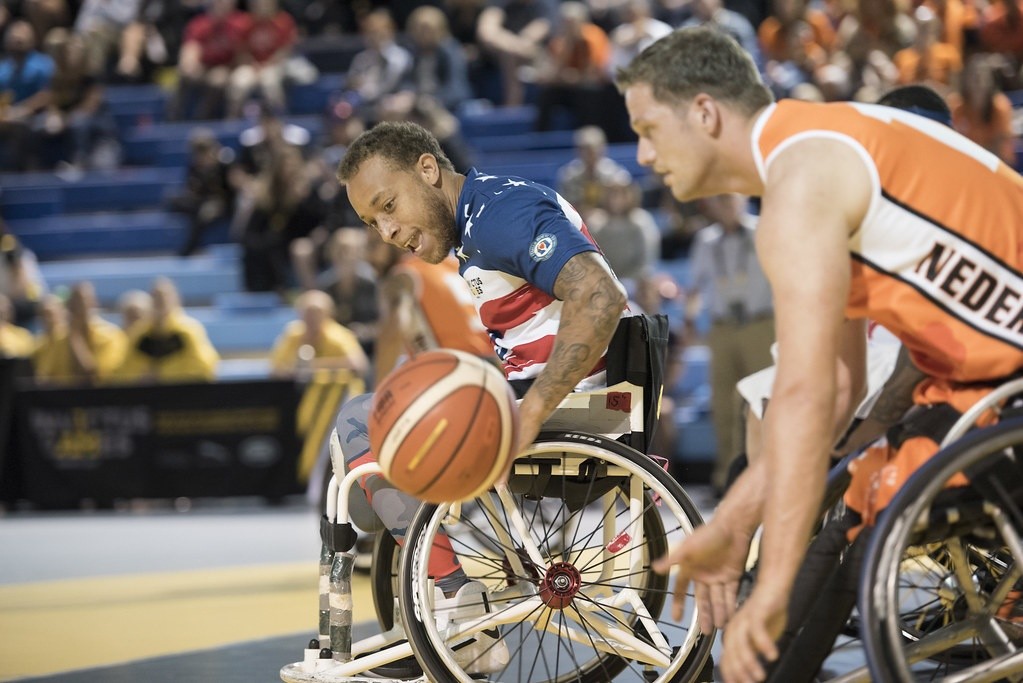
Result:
[354,582,510,681]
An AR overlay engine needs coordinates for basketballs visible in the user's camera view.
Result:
[367,345,521,505]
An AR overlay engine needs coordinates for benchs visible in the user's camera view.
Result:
[0,14,678,391]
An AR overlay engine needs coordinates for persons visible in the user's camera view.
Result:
[333,121,628,676]
[0,0,1023,509]
[614,25,1023,683]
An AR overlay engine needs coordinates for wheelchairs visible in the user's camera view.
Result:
[809,375,1023,682]
[279,314,719,683]
